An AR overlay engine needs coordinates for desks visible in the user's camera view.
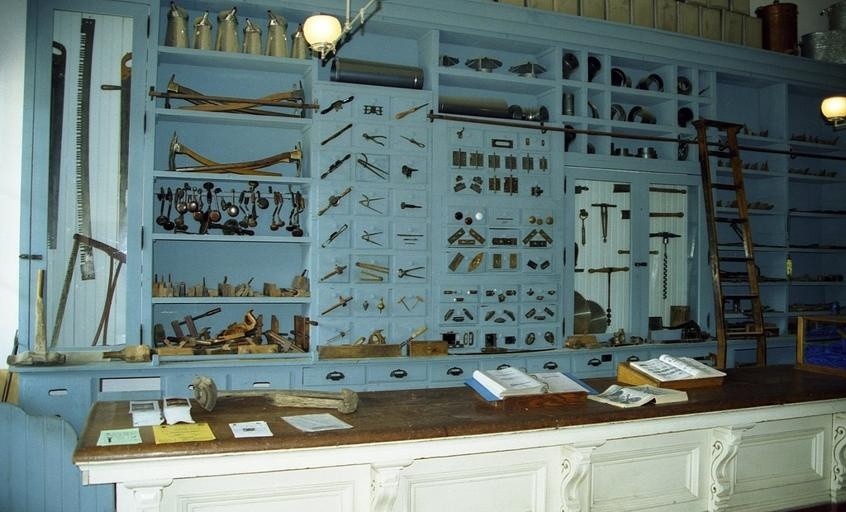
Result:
[70,363,846,510]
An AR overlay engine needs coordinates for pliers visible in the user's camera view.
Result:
[399,267,425,279]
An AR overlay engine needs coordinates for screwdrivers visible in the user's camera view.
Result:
[400,134,426,148]
[395,103,429,120]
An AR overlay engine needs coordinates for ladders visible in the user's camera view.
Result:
[692,119,767,369]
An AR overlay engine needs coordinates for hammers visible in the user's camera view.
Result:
[6,270,65,365]
[398,295,423,311]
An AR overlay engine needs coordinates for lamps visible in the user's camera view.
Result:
[304,14,343,61]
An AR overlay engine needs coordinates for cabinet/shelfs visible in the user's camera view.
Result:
[18,340,737,395]
[420,30,718,164]
[143,2,322,364]
[687,65,846,334]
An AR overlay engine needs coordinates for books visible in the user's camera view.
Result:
[746,323,779,339]
[650,328,682,340]
[670,305,689,327]
[630,354,727,382]
[464,367,599,401]
[587,384,688,408]
